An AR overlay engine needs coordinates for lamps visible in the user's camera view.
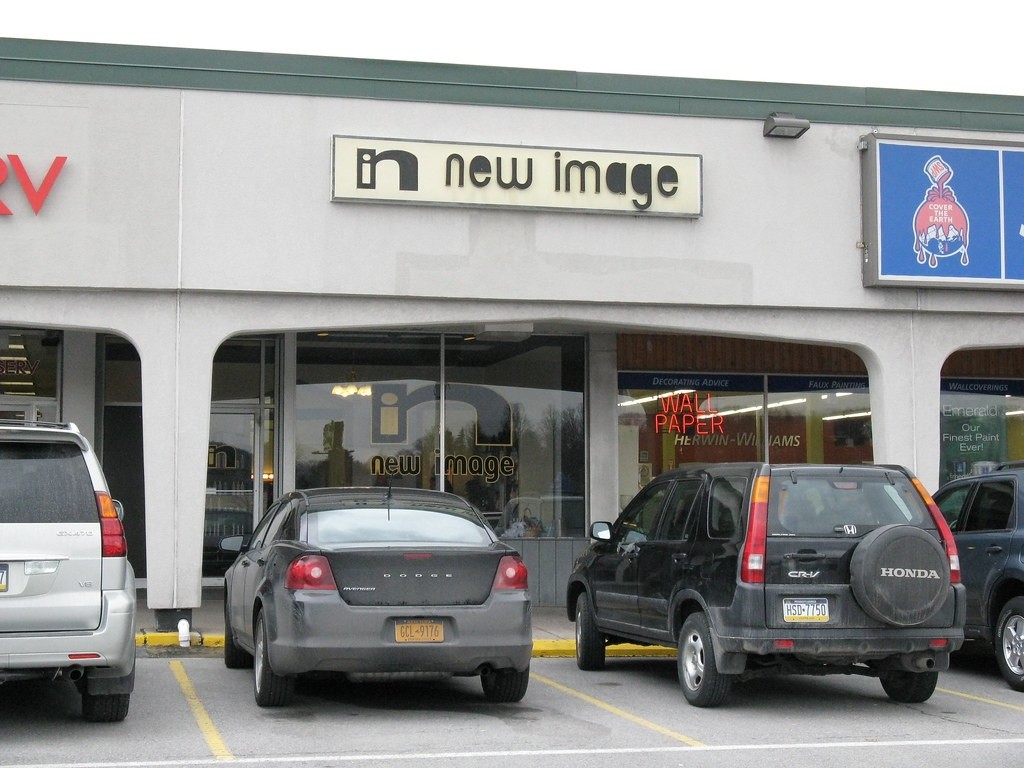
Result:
[474,322,536,342]
[331,371,374,398]
[249,419,275,482]
[763,111,810,140]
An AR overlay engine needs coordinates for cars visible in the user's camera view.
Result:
[219,487,533,708]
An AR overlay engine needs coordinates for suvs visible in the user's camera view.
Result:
[0,418,138,723]
[911,460,1024,693]
[565,460,969,708]
